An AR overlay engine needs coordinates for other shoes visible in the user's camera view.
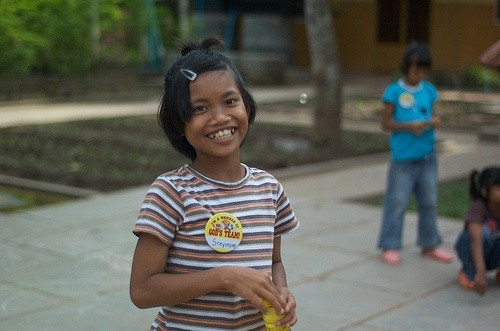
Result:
[423,248,454,263]
[381,248,401,266]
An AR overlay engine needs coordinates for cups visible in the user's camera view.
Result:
[260,297,292,331]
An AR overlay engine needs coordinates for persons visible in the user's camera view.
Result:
[126,37,300,331]
[376,44,456,264]
[454,164,500,295]
